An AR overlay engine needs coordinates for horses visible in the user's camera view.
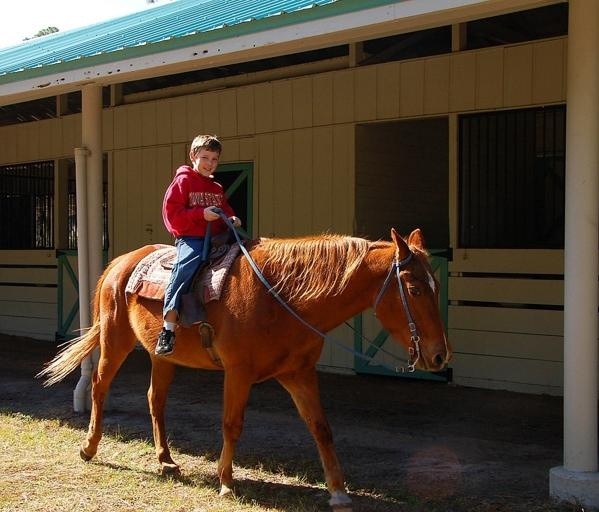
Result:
[36,226,454,512]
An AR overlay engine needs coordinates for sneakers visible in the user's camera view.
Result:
[154,328,175,355]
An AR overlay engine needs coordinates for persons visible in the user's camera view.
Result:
[154,135,244,355]
